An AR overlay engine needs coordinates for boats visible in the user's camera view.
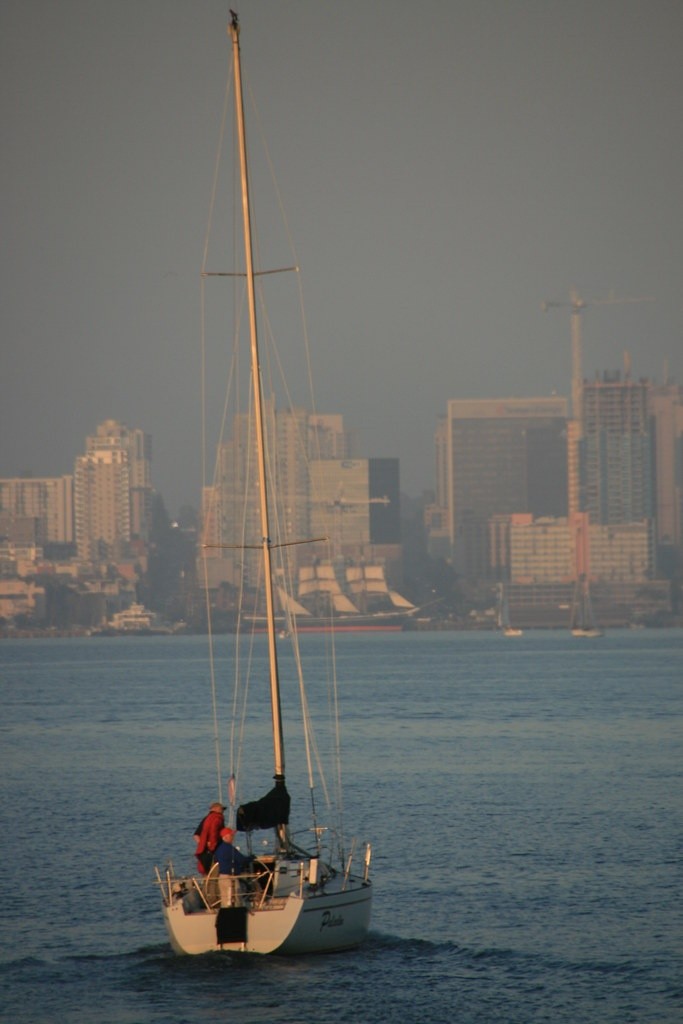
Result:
[245,549,420,632]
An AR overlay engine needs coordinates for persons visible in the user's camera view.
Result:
[215,828,255,875]
[193,801,226,875]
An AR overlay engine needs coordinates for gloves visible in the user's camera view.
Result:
[248,854,257,861]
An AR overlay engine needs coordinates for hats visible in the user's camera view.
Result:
[220,827,236,837]
[208,802,227,810]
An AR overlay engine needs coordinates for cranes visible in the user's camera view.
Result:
[220,488,391,562]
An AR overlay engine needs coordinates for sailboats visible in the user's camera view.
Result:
[148,7,376,958]
